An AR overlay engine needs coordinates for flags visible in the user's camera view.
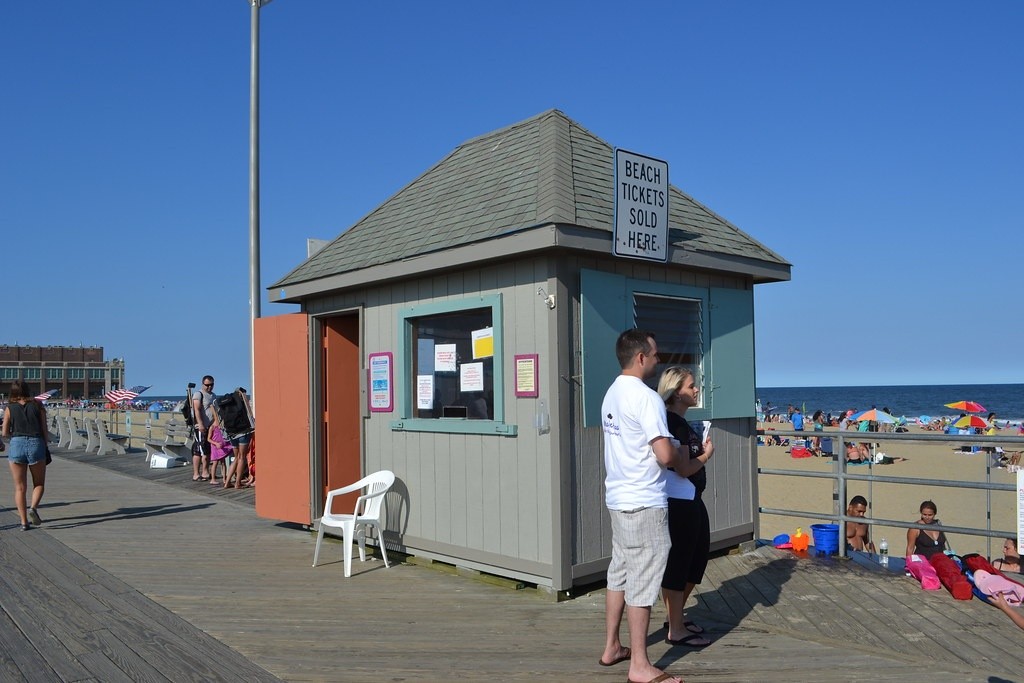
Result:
[35,389,59,400]
[105,386,149,403]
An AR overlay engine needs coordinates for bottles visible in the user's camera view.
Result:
[880,539,888,563]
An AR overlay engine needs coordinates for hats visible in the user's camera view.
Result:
[794,407,799,411]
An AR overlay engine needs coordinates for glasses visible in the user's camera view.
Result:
[203,382,215,387]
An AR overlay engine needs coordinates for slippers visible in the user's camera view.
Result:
[665,632,711,646]
[663,620,704,633]
[192,476,206,482]
[202,474,217,480]
[627,670,684,683]
[599,646,631,666]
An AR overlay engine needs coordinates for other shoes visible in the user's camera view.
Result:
[20,524,30,532]
[28,508,41,525]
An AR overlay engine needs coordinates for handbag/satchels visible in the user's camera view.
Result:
[182,391,203,426]
[44,441,52,466]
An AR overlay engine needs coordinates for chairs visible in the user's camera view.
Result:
[817,437,833,457]
[312,470,395,577]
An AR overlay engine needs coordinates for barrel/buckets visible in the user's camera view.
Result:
[811,524,840,555]
[949,426,959,437]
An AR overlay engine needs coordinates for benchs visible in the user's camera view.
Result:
[46,413,130,455]
[143,420,192,463]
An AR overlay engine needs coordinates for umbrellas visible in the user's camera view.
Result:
[953,415,993,428]
[148,403,163,413]
[943,400,987,413]
[847,408,900,432]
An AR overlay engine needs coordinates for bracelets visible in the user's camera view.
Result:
[703,453,708,465]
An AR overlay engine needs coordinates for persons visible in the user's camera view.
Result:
[906,501,946,558]
[988,592,1024,630]
[599,327,684,683]
[846,496,876,554]
[67,399,178,411]
[755,399,1024,469]
[991,538,1021,574]
[657,366,715,646]
[191,375,255,488]
[2,380,48,532]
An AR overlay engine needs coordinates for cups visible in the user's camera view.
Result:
[538,415,549,431]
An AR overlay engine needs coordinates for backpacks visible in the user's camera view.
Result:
[217,392,250,435]
